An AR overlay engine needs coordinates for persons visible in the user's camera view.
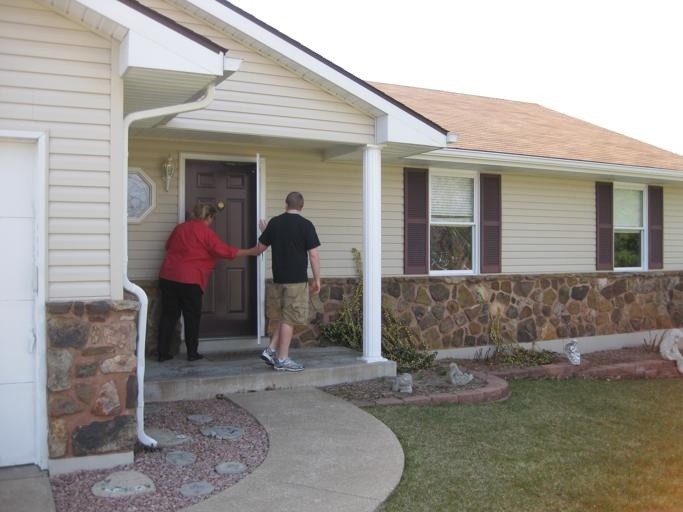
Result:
[157,201,259,362]
[256,191,321,372]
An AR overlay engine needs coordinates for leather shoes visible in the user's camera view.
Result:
[187,353,204,361]
[158,352,174,362]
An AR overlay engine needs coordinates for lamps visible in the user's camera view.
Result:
[162,152,176,192]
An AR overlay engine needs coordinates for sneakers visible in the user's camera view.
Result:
[273,357,305,372]
[260,346,278,366]
